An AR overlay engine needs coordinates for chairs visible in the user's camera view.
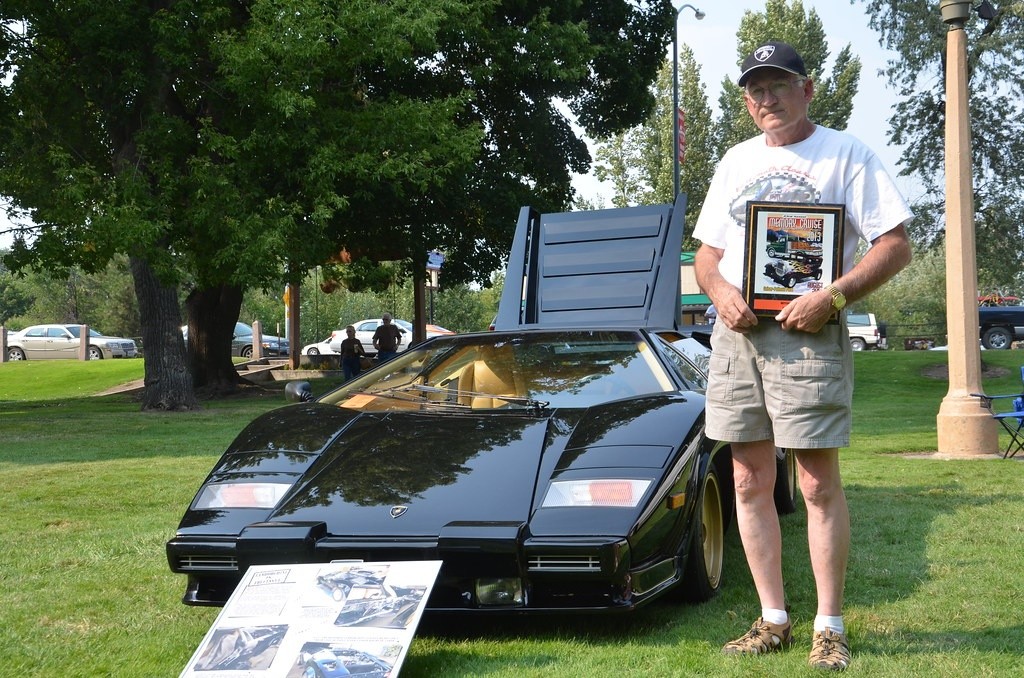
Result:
[458,343,526,407]
[970,366,1024,458]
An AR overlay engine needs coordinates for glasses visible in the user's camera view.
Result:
[744,76,807,104]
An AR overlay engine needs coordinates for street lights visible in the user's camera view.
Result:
[674,3,706,327]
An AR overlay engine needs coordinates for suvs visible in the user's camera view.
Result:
[845,313,881,351]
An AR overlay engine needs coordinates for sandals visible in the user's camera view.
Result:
[809,626,851,672]
[723,604,796,656]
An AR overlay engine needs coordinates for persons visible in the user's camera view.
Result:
[339,326,365,383]
[372,313,402,364]
[692,41,911,671]
[705,304,716,325]
[878,321,886,350]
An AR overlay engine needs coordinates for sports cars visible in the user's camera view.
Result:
[164,191,798,617]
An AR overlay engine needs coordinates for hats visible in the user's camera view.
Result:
[738,42,808,87]
[382,312,391,319]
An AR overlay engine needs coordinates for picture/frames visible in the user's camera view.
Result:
[739,200,845,322]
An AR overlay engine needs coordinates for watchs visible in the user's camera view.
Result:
[824,285,847,311]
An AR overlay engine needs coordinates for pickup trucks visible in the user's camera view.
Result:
[978,307,1024,351]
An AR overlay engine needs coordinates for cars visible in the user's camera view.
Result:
[182,320,290,358]
[6,324,137,361]
[425,323,456,337]
[329,318,433,354]
[301,335,376,356]
